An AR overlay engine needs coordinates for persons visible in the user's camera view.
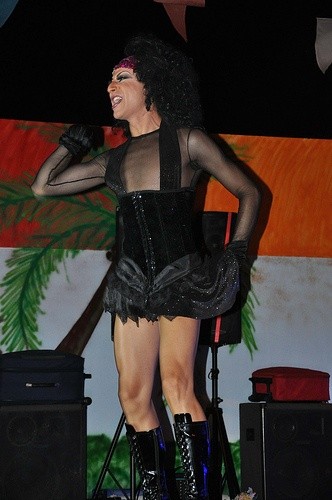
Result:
[30,32,262,500]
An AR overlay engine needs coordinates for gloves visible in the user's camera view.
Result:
[61,125,104,158]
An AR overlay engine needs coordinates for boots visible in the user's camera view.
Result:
[125,423,181,500]
[173,413,211,500]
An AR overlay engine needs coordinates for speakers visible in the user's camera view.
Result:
[0,397,92,500]
[238,402,332,500]
[193,211,241,346]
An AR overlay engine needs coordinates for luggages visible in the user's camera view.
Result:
[0,350,92,405]
[248,367,330,402]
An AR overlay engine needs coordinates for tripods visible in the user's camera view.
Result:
[92,346,238,499]
